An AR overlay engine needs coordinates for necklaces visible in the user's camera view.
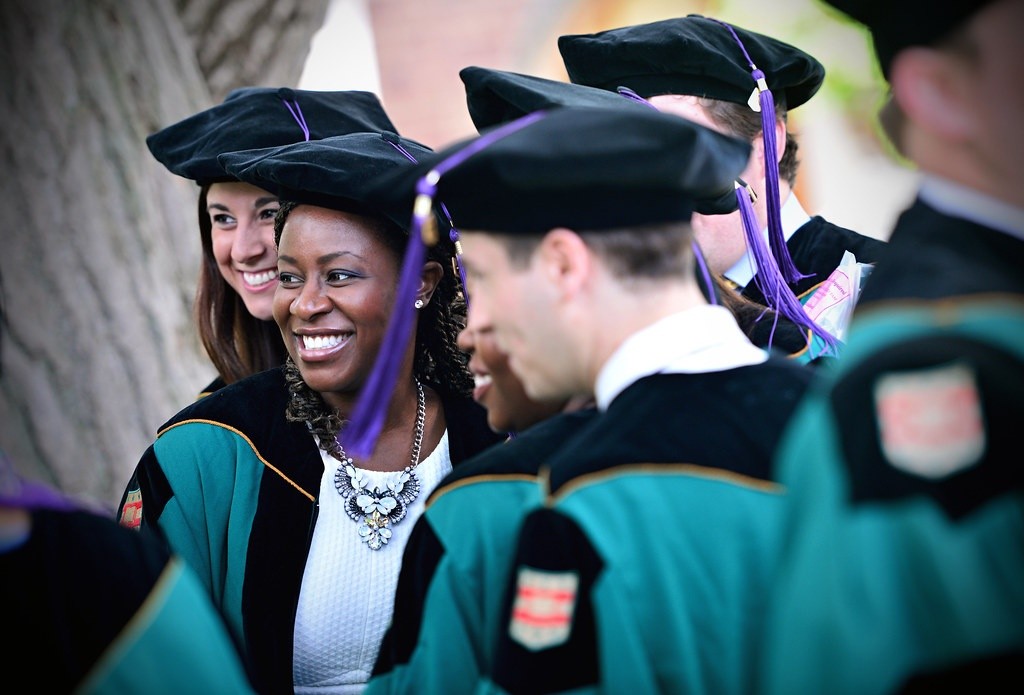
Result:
[329,377,425,551]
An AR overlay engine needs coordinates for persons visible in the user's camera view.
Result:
[559,14,886,339]
[457,64,841,359]
[104,126,509,695]
[765,0,1024,695]
[0,479,250,695]
[373,316,600,695]
[431,104,834,695]
[146,87,404,390]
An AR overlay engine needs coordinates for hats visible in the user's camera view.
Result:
[820,0,991,82]
[459,66,849,360]
[147,87,397,184]
[215,131,471,306]
[338,104,756,459]
[559,14,825,284]
